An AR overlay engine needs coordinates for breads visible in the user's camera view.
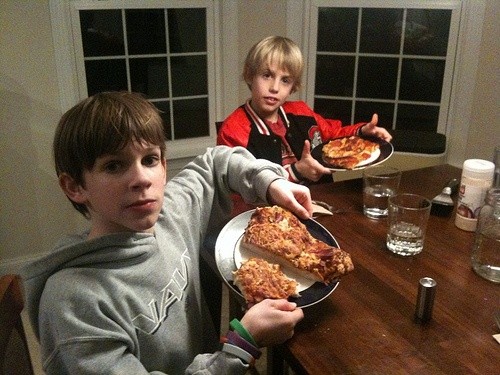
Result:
[323,134,382,173]
[242,205,354,288]
[232,258,302,305]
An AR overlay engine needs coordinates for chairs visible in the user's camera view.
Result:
[0,275,34,374]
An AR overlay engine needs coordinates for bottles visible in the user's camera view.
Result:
[455,158,496,232]
[415,277,437,321]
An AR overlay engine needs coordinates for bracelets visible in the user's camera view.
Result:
[222,317,263,370]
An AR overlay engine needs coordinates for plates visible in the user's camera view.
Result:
[308,134,394,171]
[215,204,342,309]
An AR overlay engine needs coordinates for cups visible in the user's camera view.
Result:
[365,167,401,220]
[385,193,429,256]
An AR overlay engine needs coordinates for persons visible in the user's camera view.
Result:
[18,87,315,374]
[217,35,393,219]
